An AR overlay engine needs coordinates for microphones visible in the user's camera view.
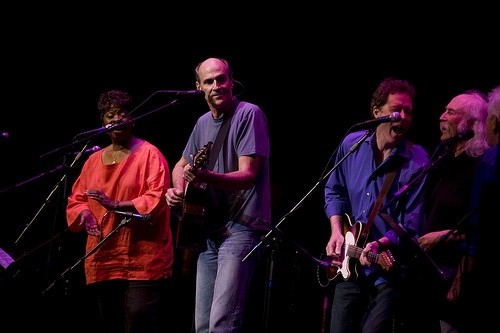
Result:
[112,210,151,220]
[160,90,206,98]
[439,129,474,145]
[356,111,401,127]
[74,144,101,155]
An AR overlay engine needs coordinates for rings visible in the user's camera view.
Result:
[97,193,101,198]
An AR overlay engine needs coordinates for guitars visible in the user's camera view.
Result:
[326,213,395,284]
[176,142,209,250]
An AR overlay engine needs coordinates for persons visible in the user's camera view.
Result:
[165,58,272,333]
[418,88,500,333]
[324,80,431,333]
[66,90,173,333]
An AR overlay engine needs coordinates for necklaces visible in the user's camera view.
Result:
[112,144,125,164]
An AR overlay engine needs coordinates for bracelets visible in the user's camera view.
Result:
[116,198,120,210]
[375,239,384,253]
